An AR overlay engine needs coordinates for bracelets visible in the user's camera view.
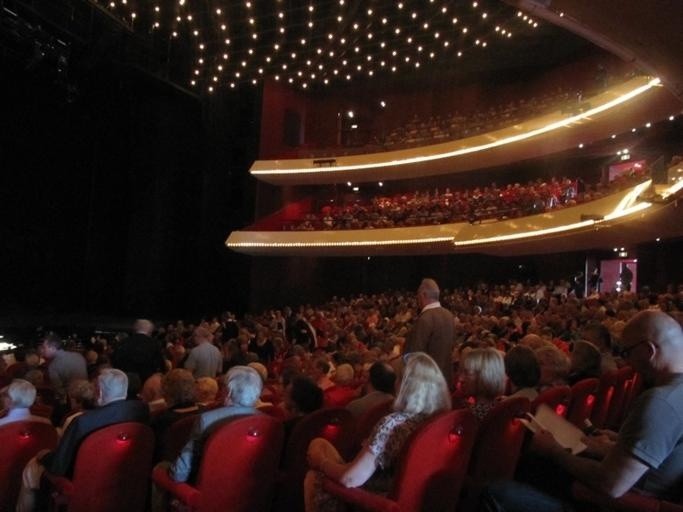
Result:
[317,455,330,475]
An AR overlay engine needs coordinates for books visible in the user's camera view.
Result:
[516,403,589,455]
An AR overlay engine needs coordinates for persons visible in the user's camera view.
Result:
[401,278,454,392]
[281,288,401,420]
[16,368,149,512]
[373,88,581,144]
[296,174,621,230]
[588,266,603,292]
[575,287,599,346]
[503,279,574,405]
[117,317,166,381]
[152,365,264,511]
[619,261,633,290]
[154,320,188,368]
[629,278,682,324]
[56,380,97,447]
[151,369,203,464]
[188,307,281,408]
[600,285,628,370]
[303,353,453,512]
[41,330,128,386]
[440,280,509,441]
[595,62,621,88]
[1,332,54,423]
[478,310,682,512]
[568,341,606,393]
[569,271,583,296]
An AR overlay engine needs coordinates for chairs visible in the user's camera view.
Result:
[570,377,602,427]
[150,413,288,512]
[289,407,353,487]
[167,414,199,458]
[470,395,529,478]
[261,393,281,403]
[321,386,363,405]
[574,480,682,512]
[530,384,572,423]
[601,368,621,424]
[611,364,639,423]
[29,404,54,419]
[355,399,395,444]
[321,408,479,512]
[257,402,283,416]
[0,417,58,512]
[37,421,157,512]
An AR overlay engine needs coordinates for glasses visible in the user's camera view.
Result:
[621,340,658,359]
